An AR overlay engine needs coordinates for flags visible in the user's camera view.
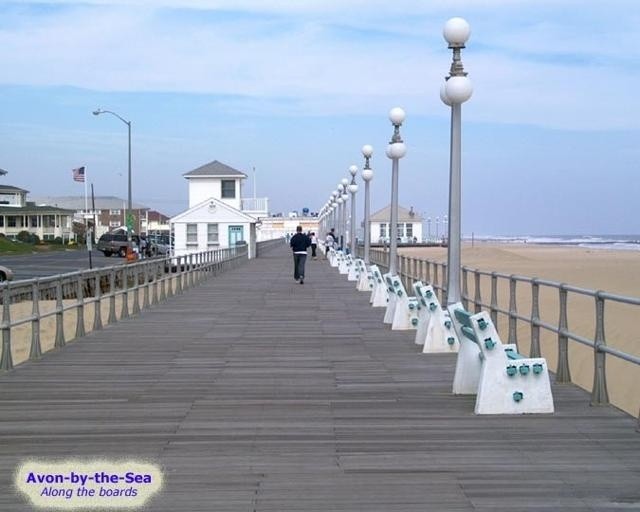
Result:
[71,166,85,183]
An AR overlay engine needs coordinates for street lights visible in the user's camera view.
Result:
[426,215,448,241]
[117,170,126,229]
[360,144,374,265]
[346,164,359,259]
[316,177,348,257]
[93,109,135,264]
[385,108,406,276]
[440,15,474,311]
[252,165,257,199]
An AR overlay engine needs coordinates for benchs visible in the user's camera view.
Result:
[449,304,557,417]
[317,240,466,355]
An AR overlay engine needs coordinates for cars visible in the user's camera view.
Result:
[0,265,15,284]
[95,232,174,257]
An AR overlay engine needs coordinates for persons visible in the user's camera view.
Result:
[139,237,147,260]
[145,237,151,258]
[289,225,312,286]
[325,227,336,258]
[132,238,137,253]
[309,231,319,257]
[320,232,334,259]
